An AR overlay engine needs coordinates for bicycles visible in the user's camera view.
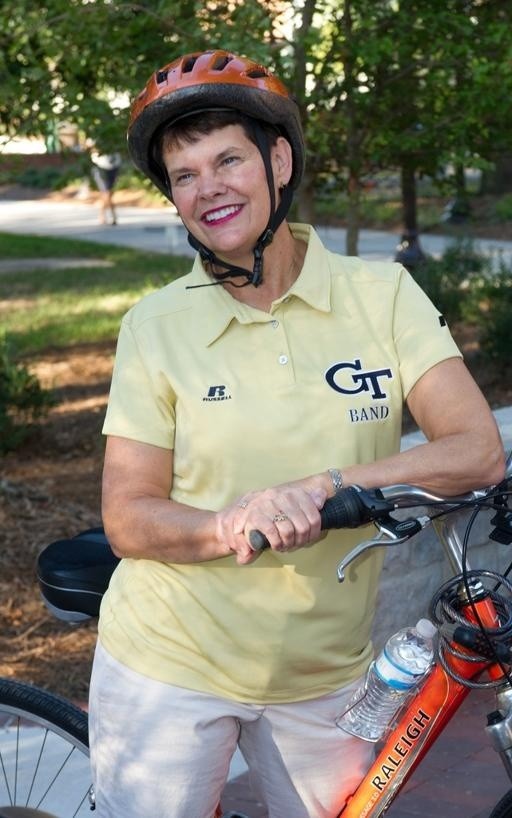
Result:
[1,451,511,817]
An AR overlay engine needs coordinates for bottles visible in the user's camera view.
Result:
[341,614,439,740]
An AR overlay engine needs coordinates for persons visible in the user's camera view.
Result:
[85,46,507,817]
[90,149,124,226]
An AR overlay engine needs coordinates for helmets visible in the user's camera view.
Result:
[125,49,308,192]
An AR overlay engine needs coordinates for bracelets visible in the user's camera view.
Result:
[327,466,344,489]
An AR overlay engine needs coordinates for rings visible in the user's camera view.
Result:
[272,511,288,521]
[237,499,249,507]
